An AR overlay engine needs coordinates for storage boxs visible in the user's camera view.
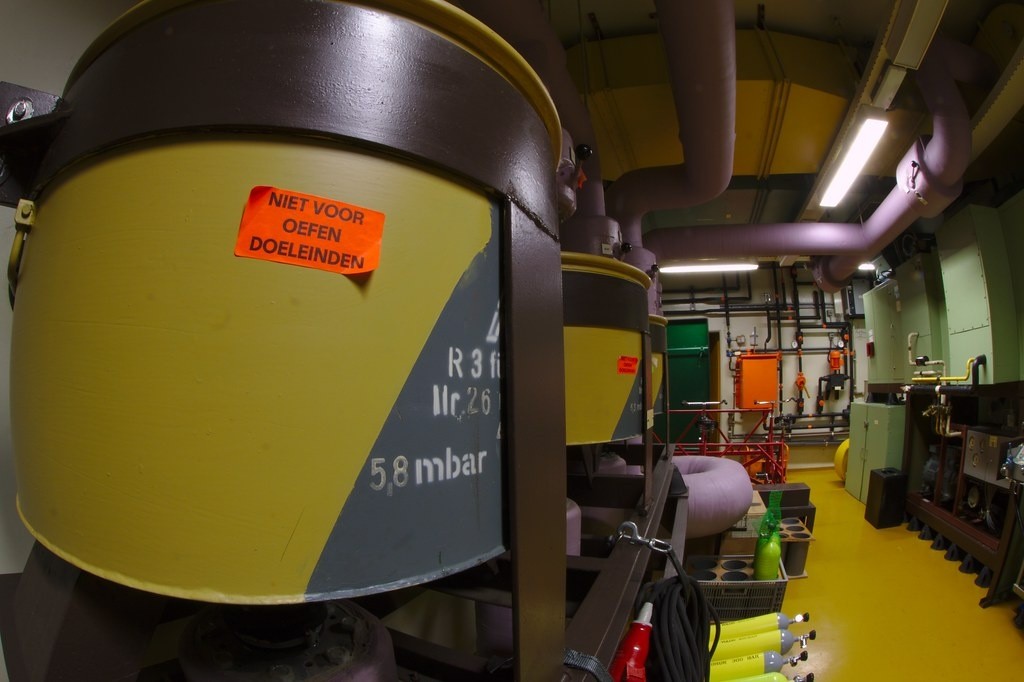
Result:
[686,554,789,621]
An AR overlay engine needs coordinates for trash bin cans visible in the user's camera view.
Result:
[864,467,907,530]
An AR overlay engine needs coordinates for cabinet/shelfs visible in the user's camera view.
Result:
[845,402,906,504]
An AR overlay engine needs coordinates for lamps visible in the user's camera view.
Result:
[820,104,889,206]
[659,259,759,273]
[885,0,949,70]
[779,218,817,267]
